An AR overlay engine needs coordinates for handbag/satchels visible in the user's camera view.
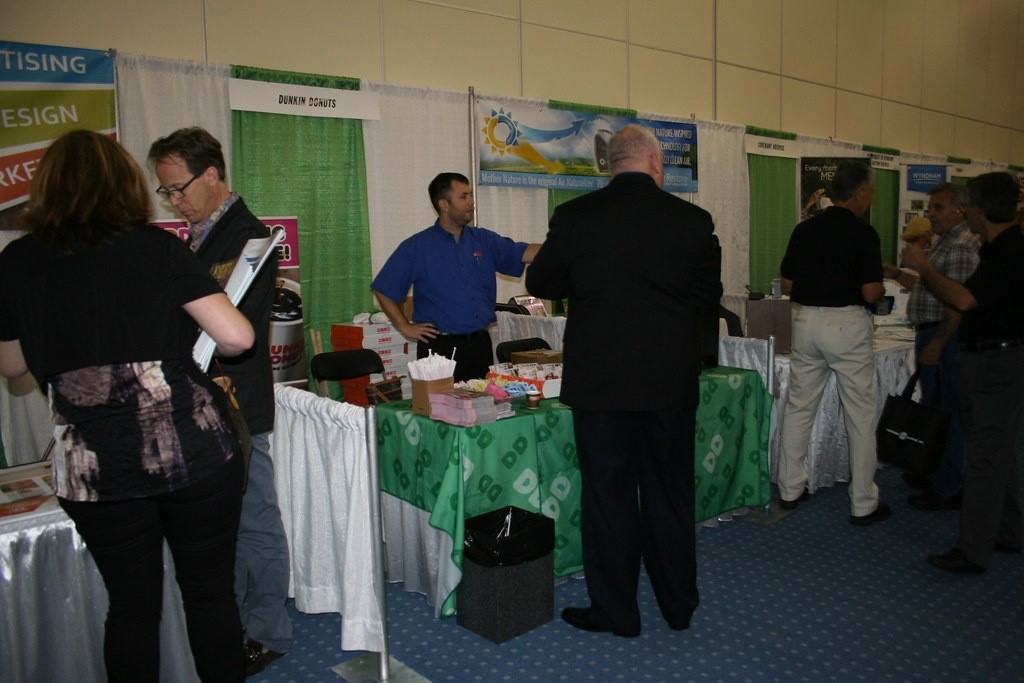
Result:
[213,376,252,493]
[876,365,947,472]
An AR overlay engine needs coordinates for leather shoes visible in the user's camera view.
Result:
[562,607,640,637]
[669,584,699,630]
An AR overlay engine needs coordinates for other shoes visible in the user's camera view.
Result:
[901,473,935,490]
[777,486,809,509]
[908,471,975,510]
[850,502,891,524]
[241,637,286,675]
[927,547,987,573]
[993,541,1020,553]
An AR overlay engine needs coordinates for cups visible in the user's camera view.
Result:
[268,278,305,382]
[525,391,541,409]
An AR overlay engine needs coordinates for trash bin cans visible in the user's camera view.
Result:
[457,506,556,642]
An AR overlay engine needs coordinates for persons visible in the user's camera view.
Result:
[777,160,892,524]
[899,171,1024,576]
[145,127,295,679]
[1,127,256,682]
[369,173,542,381]
[882,183,979,512]
[526,123,722,636]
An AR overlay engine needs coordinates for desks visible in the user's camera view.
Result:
[747,296,792,354]
[374,364,773,620]
[0,457,202,683]
[768,328,922,501]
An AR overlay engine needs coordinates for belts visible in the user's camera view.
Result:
[960,339,1023,353]
[912,322,942,331]
[438,330,485,341]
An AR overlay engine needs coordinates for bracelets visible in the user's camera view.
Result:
[894,270,901,279]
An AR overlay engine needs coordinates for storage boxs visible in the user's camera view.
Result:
[331,322,418,405]
[412,377,454,417]
[486,350,562,399]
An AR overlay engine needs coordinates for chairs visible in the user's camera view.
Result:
[310,349,388,399]
[496,337,551,363]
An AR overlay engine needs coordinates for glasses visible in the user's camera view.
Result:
[156,167,208,200]
